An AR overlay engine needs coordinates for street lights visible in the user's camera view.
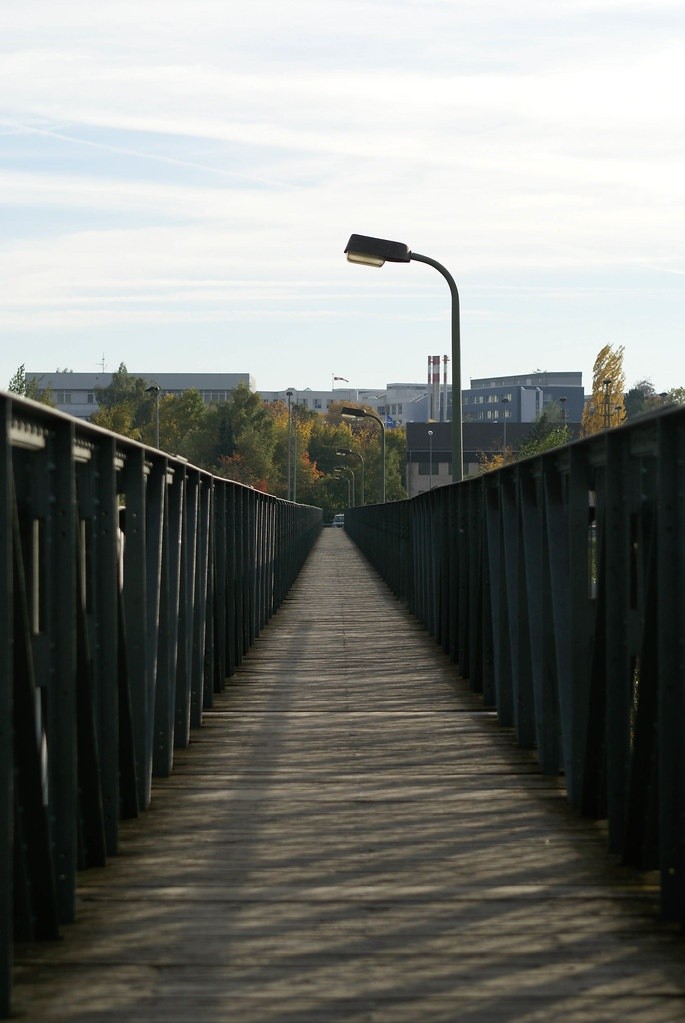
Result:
[559,396,567,445]
[501,396,510,462]
[336,448,365,506]
[335,474,350,507]
[335,466,355,507]
[603,379,613,428]
[341,407,387,504]
[343,234,466,483]
[285,391,293,502]
[614,406,622,428]
[427,430,433,491]
[144,385,160,452]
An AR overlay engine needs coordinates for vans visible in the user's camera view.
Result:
[333,514,345,528]
